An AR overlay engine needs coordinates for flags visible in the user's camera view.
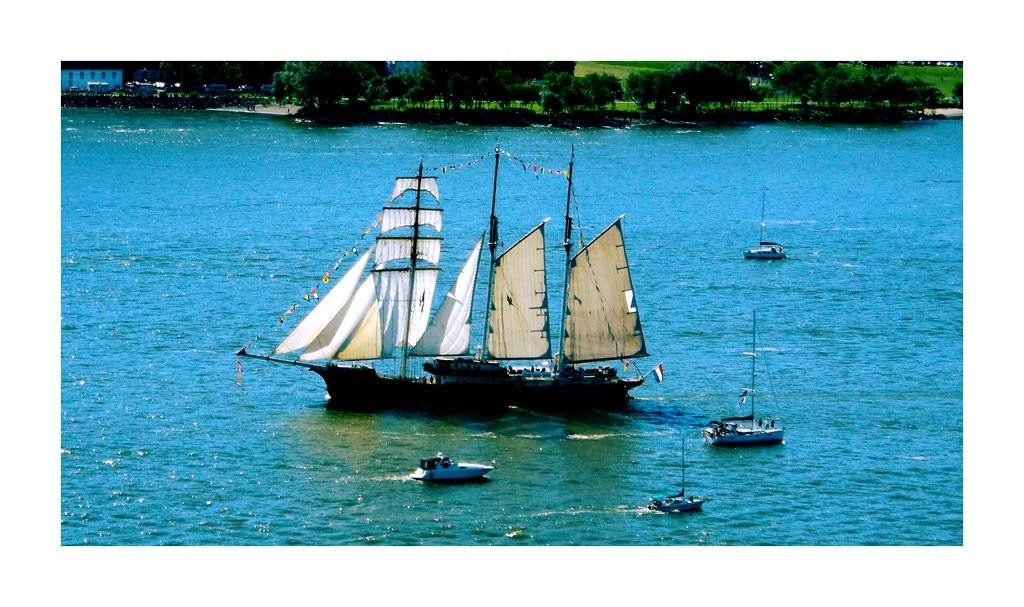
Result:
[652,363,663,383]
[737,390,747,408]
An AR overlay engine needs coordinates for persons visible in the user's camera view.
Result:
[507,364,549,375]
[754,419,757,429]
[758,418,762,428]
[765,418,769,429]
[771,419,775,428]
[422,375,434,384]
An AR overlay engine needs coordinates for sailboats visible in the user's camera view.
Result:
[742,189,787,260]
[703,309,786,446]
[647,438,705,513]
[231,136,653,417]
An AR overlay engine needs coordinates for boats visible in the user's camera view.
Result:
[408,451,495,481]
